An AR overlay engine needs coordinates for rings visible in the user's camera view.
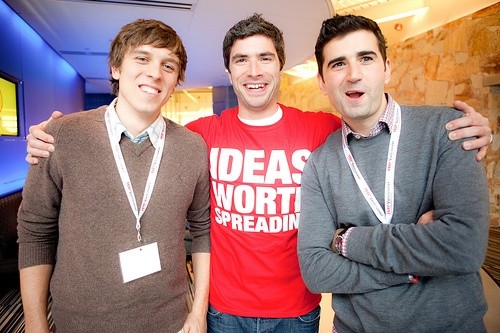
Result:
[489,133,494,142]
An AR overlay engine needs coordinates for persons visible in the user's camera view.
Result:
[297,13,489,333]
[25,13,494,333]
[16,20,210,332]
